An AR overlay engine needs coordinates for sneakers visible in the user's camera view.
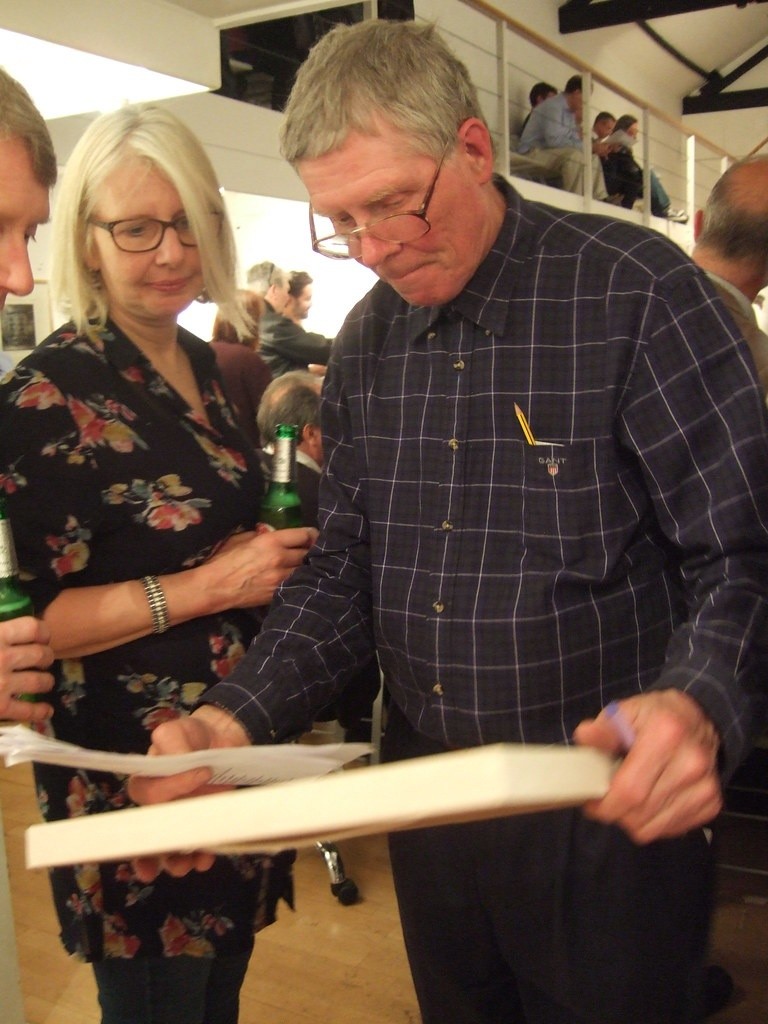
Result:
[662,209,688,222]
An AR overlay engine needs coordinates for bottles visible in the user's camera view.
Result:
[254,421,305,537]
[0,492,55,728]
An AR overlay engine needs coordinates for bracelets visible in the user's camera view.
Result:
[141,577,171,631]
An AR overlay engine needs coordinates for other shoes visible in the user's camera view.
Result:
[605,193,626,204]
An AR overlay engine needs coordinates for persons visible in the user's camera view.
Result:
[0,68,383,1024]
[514,76,768,399]
[126,19,766,1023]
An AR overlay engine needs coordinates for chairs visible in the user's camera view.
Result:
[509,134,556,186]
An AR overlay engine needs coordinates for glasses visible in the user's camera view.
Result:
[88,209,225,255]
[307,133,453,261]
